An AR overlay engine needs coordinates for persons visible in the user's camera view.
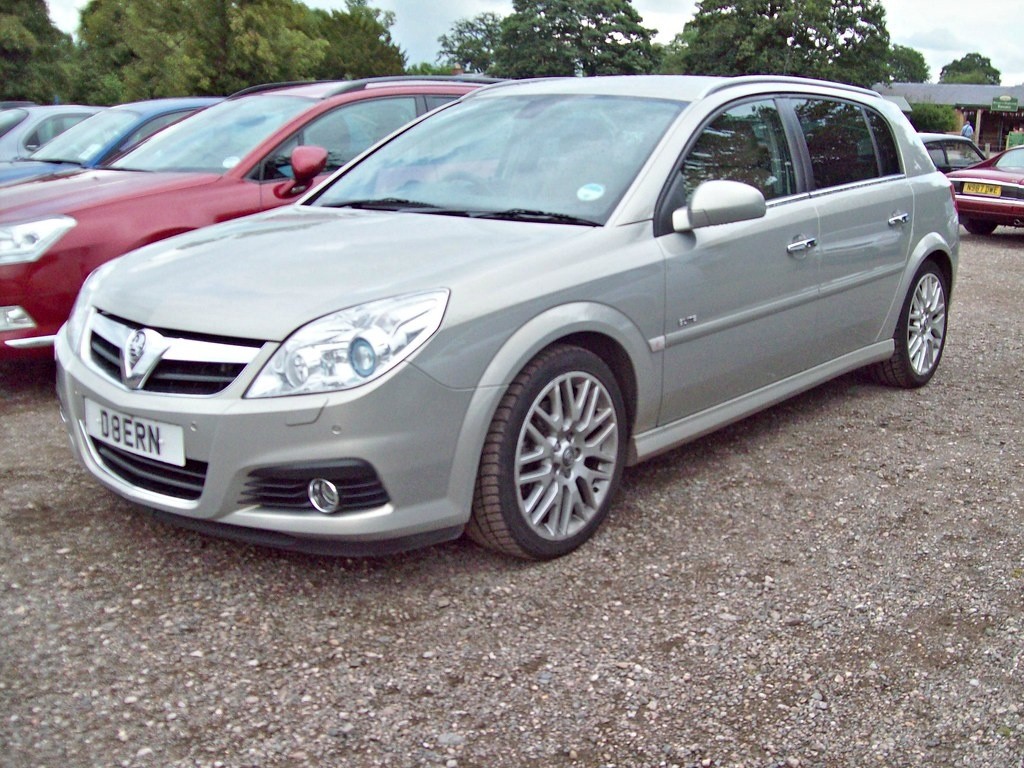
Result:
[1013,126,1018,132]
[1019,128,1024,132]
[959,122,973,149]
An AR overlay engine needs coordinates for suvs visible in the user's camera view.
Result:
[0,77,509,356]
[54,76,959,559]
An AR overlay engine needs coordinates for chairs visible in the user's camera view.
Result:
[686,121,774,200]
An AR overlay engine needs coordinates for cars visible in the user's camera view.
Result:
[0,98,414,188]
[944,145,1024,235]
[918,132,987,173]
